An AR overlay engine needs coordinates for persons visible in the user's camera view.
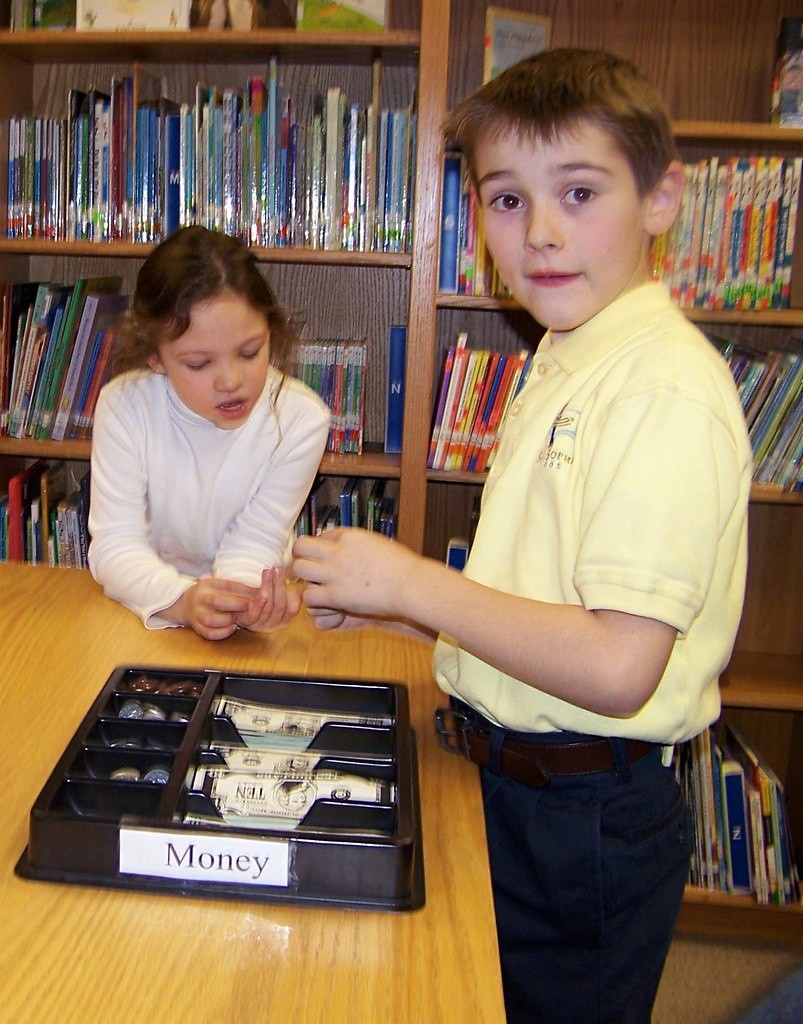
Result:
[87,227,329,642]
[293,47,753,1024]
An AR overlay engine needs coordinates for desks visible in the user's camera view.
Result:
[0,560,507,1024]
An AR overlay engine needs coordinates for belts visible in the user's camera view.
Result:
[435,694,659,788]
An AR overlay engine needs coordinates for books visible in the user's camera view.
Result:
[428,145,803,575]
[1,277,408,581]
[663,719,803,903]
[1,61,419,255]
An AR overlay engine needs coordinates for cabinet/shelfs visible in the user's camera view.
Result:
[0,0,803,953]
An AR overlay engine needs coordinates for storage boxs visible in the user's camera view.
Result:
[14,663,427,911]
